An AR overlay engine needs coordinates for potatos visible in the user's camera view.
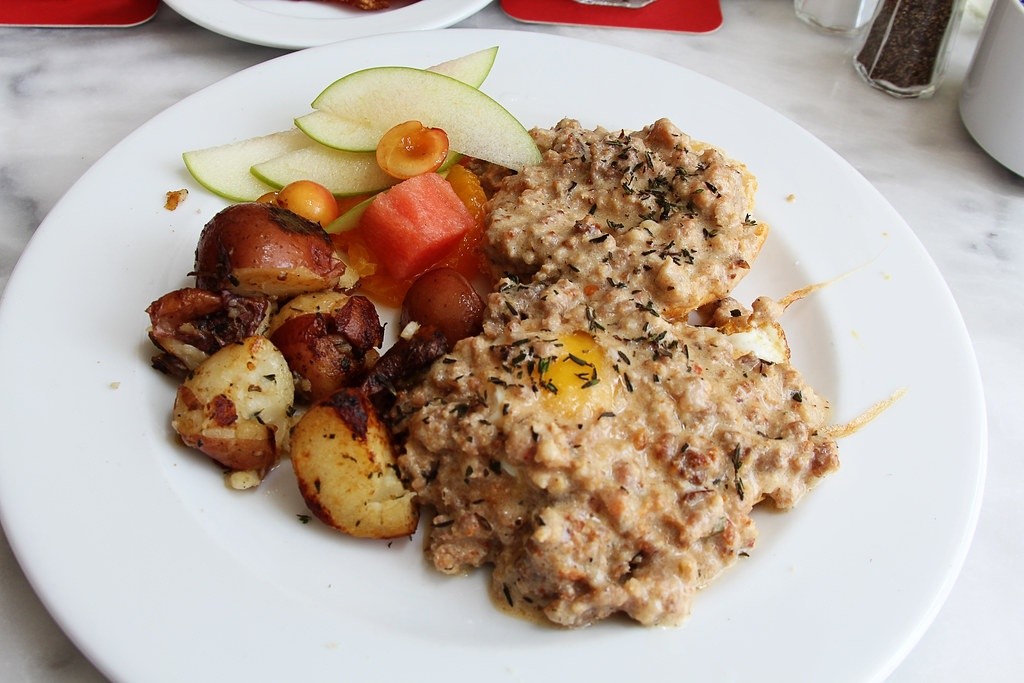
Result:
[146,203,487,540]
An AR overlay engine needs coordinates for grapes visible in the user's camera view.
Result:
[377,119,450,179]
[278,180,339,229]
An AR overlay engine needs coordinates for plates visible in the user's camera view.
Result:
[499,0,724,35]
[161,0,496,51]
[0,0,159,28]
[1,27,989,683]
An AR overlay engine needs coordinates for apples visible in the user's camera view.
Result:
[181,46,542,206]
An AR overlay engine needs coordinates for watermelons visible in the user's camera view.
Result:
[358,171,475,278]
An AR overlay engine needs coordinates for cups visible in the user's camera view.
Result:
[794,0,883,37]
[957,0,1024,181]
[853,0,967,99]
